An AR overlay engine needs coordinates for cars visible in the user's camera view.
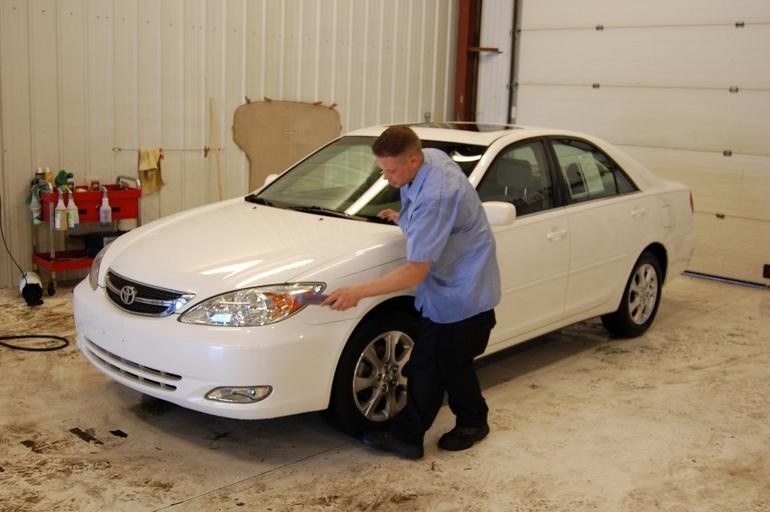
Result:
[73,122,693,437]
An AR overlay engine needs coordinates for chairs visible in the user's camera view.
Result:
[479,158,604,217]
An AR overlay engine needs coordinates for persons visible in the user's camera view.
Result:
[321,124,499,459]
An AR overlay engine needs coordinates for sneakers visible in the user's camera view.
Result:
[363,431,424,458]
[438,424,489,451]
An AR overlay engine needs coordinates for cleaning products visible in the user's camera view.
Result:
[54,187,67,230]
[28,184,44,227]
[66,188,80,229]
[99,185,113,227]
